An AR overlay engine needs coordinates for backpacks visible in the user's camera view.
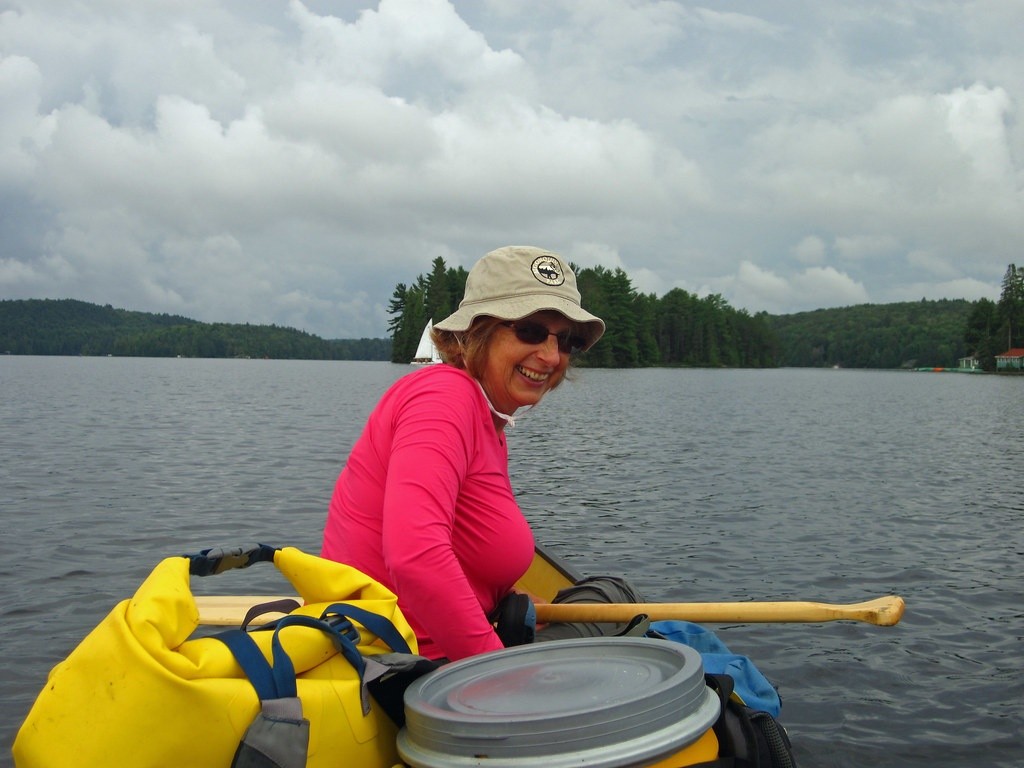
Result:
[705,672,797,768]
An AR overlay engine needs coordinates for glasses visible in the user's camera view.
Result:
[502,321,575,354]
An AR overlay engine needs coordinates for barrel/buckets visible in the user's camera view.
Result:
[396,637,722,768]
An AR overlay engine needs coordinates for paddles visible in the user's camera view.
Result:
[188,592,906,635]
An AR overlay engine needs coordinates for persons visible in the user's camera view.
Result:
[320,245,577,658]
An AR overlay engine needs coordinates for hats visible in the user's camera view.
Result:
[434,246,606,354]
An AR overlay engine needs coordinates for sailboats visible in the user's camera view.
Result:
[409,317,443,366]
